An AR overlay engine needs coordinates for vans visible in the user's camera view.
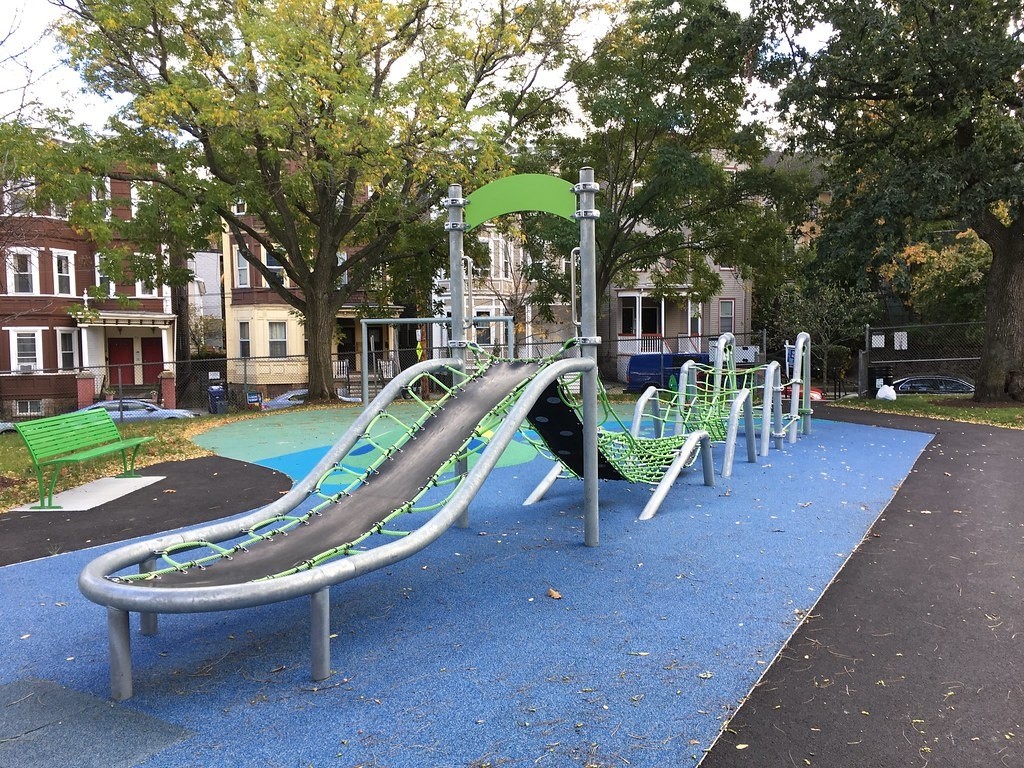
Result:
[622,352,710,395]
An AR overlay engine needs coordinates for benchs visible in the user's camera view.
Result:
[13,408,156,509]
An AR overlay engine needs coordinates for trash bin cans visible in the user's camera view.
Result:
[867,365,893,400]
[208,386,228,414]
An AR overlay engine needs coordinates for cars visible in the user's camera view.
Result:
[261,389,362,412]
[696,367,825,402]
[892,375,976,395]
[59,398,206,422]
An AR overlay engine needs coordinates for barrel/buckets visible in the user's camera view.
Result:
[217,400,228,414]
[868,365,893,398]
